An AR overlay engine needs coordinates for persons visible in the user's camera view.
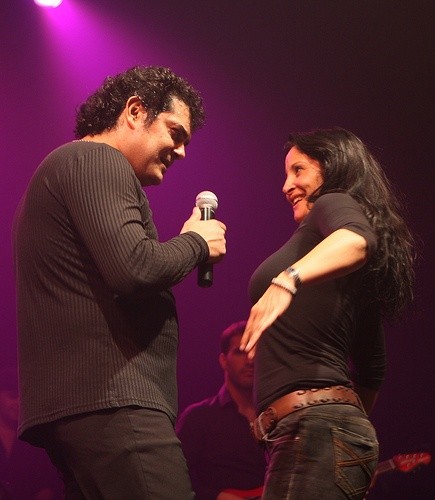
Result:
[238,128,420,500]
[174,320,378,500]
[14,66,228,500]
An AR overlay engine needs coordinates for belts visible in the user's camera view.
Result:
[252,385,362,441]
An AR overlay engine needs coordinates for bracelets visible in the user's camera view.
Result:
[271,267,302,295]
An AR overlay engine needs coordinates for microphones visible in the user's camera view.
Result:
[196,191,218,288]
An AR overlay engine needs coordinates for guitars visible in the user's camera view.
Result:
[217,452,431,500]
